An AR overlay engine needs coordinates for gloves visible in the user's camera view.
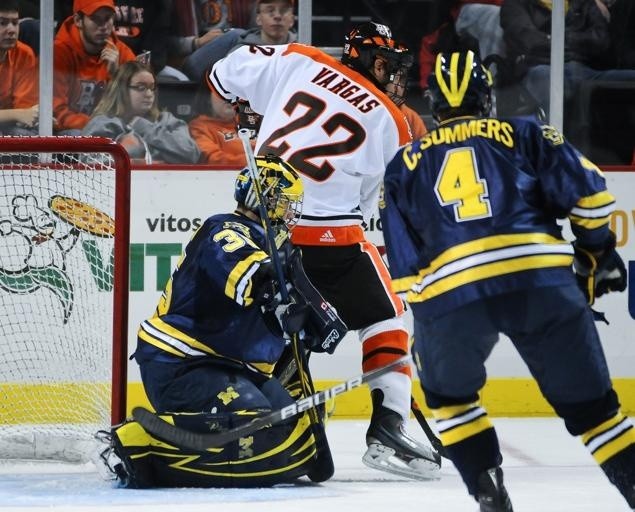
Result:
[570,230,627,305]
[258,279,313,340]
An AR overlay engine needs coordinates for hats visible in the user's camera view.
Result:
[74,0,117,15]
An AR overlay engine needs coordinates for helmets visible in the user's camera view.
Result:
[233,156,304,240]
[428,47,493,123]
[344,21,409,105]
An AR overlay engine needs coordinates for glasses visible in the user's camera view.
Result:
[129,85,159,93]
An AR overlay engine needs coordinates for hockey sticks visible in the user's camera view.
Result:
[131,353,413,454]
[237,126,336,482]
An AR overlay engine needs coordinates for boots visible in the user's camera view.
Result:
[478,467,516,512]
[361,388,443,472]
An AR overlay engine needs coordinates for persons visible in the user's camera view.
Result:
[1,0,39,138]
[89,157,334,478]
[378,49,635,511]
[207,19,441,468]
[445,1,635,164]
[53,2,297,164]
